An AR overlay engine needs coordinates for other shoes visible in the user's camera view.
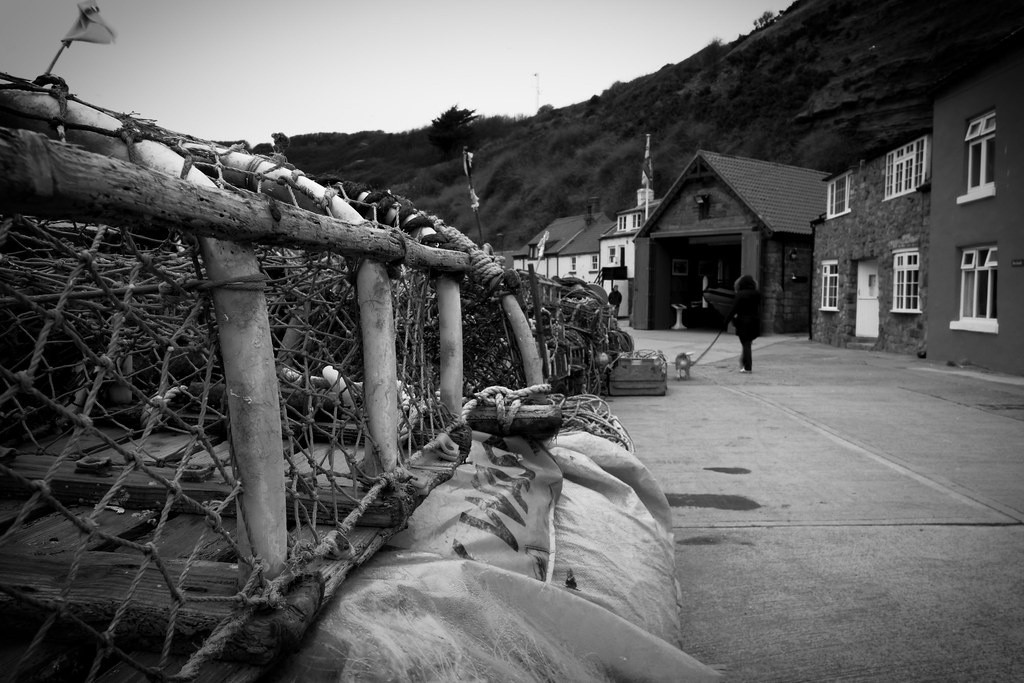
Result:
[740,368,751,373]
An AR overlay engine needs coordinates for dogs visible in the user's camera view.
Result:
[675,353,691,381]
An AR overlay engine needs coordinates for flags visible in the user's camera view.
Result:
[642,140,654,184]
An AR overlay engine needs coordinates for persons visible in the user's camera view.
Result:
[608,285,623,319]
[729,275,764,373]
